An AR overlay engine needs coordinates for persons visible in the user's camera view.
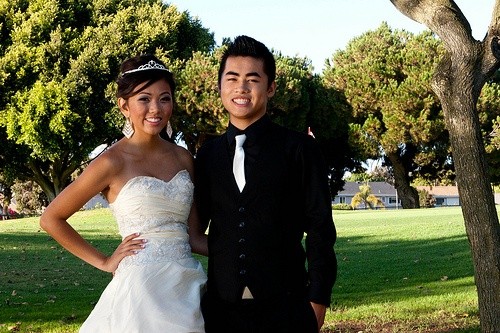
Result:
[73,178,76,182]
[194,33,338,333]
[67,175,73,183]
[426,195,436,208]
[41,197,50,210]
[39,54,204,333]
[0,214,3,219]
[65,178,70,187]
[9,208,15,214]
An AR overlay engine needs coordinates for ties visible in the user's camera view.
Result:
[233,135,251,193]
